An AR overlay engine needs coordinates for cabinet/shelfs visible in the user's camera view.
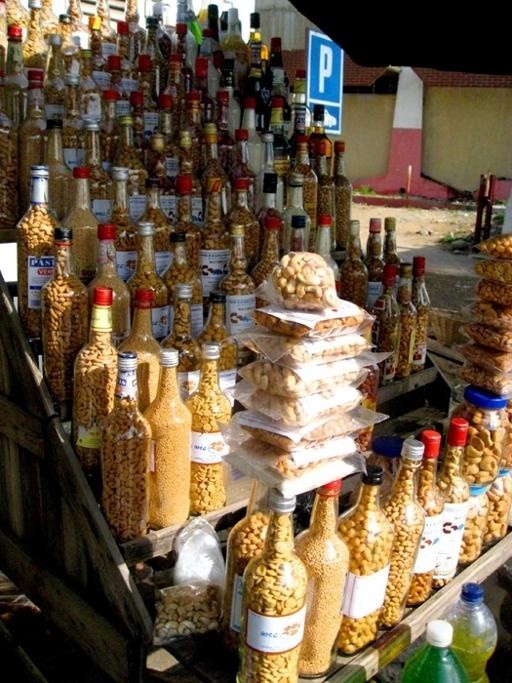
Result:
[1,234,512,682]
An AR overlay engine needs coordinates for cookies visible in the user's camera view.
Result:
[0,0,512,683]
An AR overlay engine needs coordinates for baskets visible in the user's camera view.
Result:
[430,309,470,346]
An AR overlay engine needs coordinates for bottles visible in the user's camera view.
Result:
[218,379,511,681]
[1,0,434,540]
